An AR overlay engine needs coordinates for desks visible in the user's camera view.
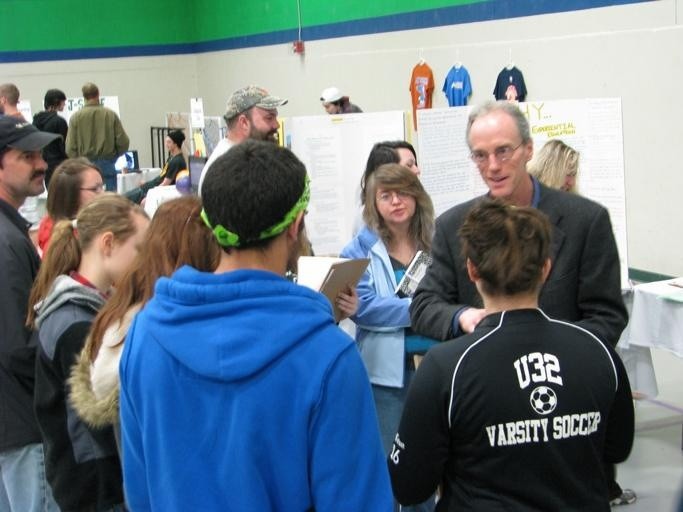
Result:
[633,275,683,413]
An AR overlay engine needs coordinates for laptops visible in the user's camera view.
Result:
[115,150,142,173]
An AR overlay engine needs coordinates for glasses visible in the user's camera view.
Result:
[376,190,411,202]
[469,139,525,163]
[79,184,107,194]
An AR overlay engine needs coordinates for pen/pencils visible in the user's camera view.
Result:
[404,272,421,286]
[669,284,682,287]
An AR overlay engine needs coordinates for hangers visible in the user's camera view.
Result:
[416,48,426,65]
[501,41,516,72]
[450,44,462,69]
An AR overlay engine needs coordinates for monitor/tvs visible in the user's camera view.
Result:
[187,156,207,192]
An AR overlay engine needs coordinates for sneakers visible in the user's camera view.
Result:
[610,487,637,507]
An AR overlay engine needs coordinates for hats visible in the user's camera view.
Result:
[167,129,185,150]
[223,85,289,119]
[320,86,344,103]
[1,116,63,152]
